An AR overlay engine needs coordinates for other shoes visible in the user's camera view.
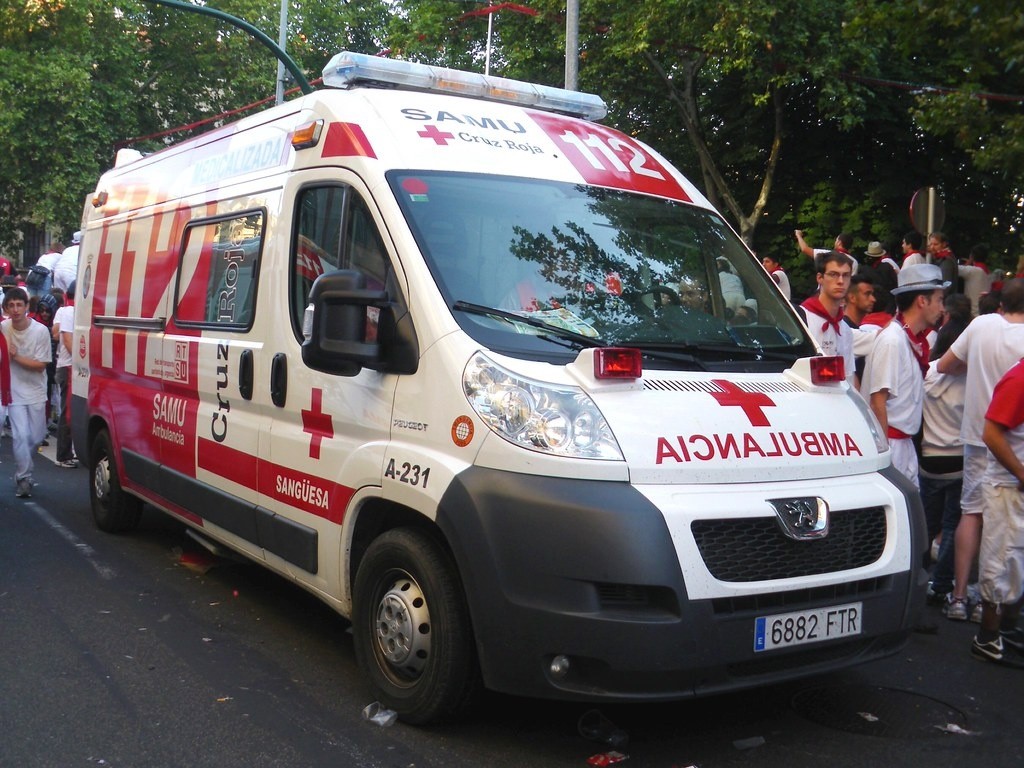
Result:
[926,580,947,607]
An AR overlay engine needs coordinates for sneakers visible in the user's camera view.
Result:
[55,459,77,468]
[971,634,1024,668]
[941,592,967,620]
[16,478,32,498]
[969,604,983,622]
[1000,626,1023,653]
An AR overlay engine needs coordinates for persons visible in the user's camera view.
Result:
[762,230,1024,666]
[496,241,634,337]
[0,231,83,499]
[660,256,759,326]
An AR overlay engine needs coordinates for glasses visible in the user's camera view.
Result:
[824,272,852,280]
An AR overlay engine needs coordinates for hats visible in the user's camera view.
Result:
[72,230,81,243]
[1,275,18,288]
[864,241,886,257]
[890,263,952,295]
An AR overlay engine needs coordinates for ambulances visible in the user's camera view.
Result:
[63,49,933,732]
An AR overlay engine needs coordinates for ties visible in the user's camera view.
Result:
[873,255,890,268]
[898,313,930,380]
[802,295,844,336]
[901,251,918,267]
